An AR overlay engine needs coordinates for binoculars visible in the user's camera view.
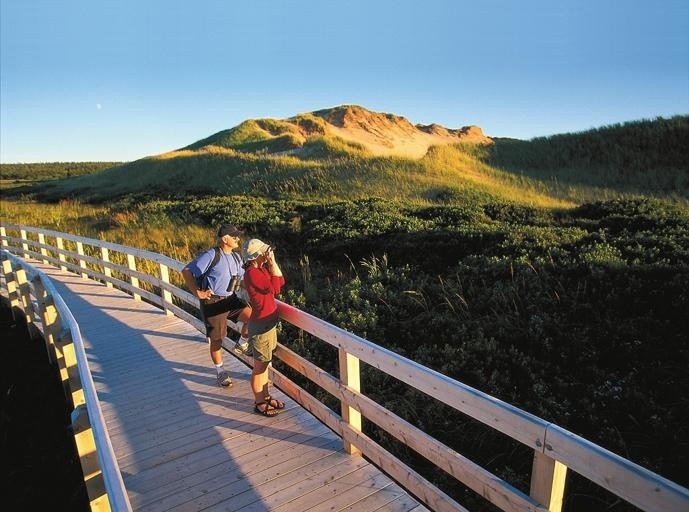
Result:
[267,245,276,251]
[226,275,241,292]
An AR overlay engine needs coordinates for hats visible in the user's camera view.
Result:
[244,239,269,260]
[218,224,244,238]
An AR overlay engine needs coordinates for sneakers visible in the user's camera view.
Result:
[235,342,253,356]
[216,371,232,387]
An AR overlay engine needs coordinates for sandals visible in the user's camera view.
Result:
[265,395,285,409]
[254,402,279,417]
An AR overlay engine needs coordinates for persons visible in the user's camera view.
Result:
[181,223,253,387]
[240,238,286,417]
